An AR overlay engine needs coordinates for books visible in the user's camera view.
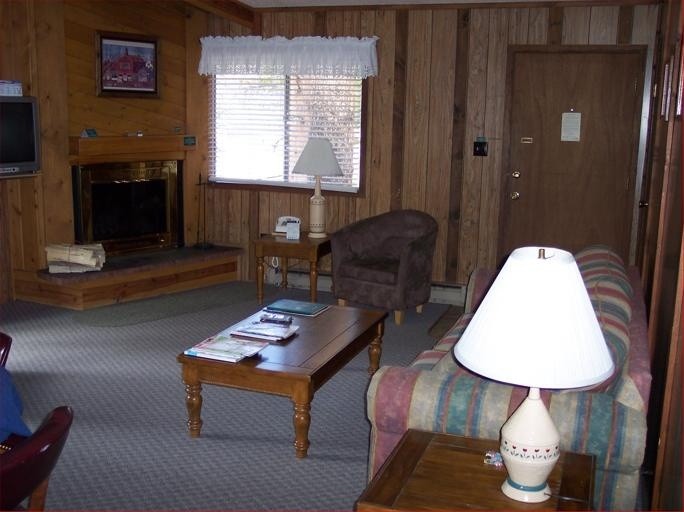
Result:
[184,299,330,363]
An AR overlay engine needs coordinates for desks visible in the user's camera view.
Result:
[354,428,599,512]
[250,226,334,306]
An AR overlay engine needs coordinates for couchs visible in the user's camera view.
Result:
[363,235,656,512]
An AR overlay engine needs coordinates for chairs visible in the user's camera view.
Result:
[0,404,77,511]
[331,208,438,326]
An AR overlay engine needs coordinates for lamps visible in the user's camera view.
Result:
[451,240,615,504]
[292,135,343,239]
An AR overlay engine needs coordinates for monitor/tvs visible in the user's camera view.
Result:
[0,96,41,176]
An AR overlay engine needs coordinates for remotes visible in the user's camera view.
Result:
[261,314,292,325]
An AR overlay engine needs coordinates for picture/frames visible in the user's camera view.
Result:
[94,27,164,100]
